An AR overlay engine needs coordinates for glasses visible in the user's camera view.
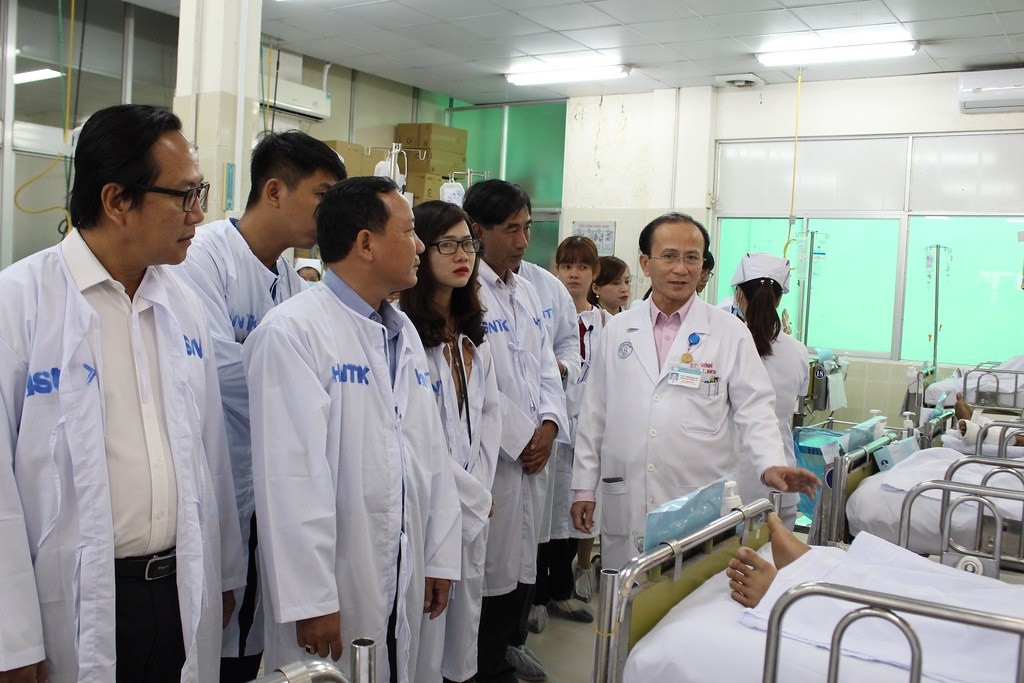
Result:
[700,271,714,278]
[649,254,706,267]
[431,238,482,255]
[136,182,211,212]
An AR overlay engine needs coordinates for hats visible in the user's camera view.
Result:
[731,252,791,293]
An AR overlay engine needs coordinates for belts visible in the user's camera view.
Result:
[115,549,177,581]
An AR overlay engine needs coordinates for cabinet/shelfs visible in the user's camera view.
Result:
[790,417,914,535]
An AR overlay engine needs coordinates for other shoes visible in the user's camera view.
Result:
[505,644,546,679]
[544,598,594,622]
[526,603,548,633]
[574,561,594,603]
[591,554,603,584]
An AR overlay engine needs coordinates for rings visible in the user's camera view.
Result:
[305,644,314,654]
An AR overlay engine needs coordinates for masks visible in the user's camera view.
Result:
[732,289,744,319]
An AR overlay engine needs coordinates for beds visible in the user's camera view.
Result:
[826,422,1024,584]
[926,410,1024,459]
[593,490,1024,683]
[915,361,1024,427]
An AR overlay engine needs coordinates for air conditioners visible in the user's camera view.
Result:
[260,72,332,124]
[957,67,1024,114]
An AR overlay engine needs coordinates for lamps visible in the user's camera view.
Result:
[754,39,922,68]
[502,64,632,87]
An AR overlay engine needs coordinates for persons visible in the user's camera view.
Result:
[671,373,677,379]
[727,511,813,609]
[570,213,824,571]
[730,252,810,534]
[0,103,631,683]
[955,392,1024,447]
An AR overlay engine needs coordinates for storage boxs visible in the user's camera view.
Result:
[324,140,362,179]
[400,173,441,207]
[398,148,468,179]
[396,123,468,155]
[362,147,388,176]
